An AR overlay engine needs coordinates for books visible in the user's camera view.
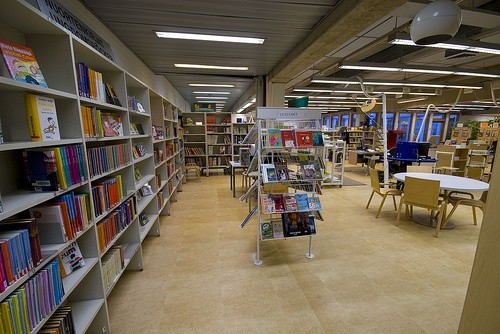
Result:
[126,81,381,248]
[97,193,139,253]
[86,141,131,180]
[0,38,55,88]
[38,304,78,334]
[92,172,129,218]
[76,59,126,106]
[1,185,93,294]
[21,143,84,195]
[24,92,61,143]
[0,240,86,334]
[80,107,125,138]
[101,243,124,293]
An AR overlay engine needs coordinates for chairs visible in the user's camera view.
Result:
[365,135,491,238]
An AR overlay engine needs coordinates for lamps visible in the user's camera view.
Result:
[409,0,463,45]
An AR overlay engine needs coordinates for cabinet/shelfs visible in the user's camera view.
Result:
[320,128,378,188]
[0,0,254,334]
[239,107,325,264]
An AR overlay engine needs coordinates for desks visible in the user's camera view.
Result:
[229,160,251,198]
[349,149,390,176]
[381,141,438,192]
[394,172,490,229]
